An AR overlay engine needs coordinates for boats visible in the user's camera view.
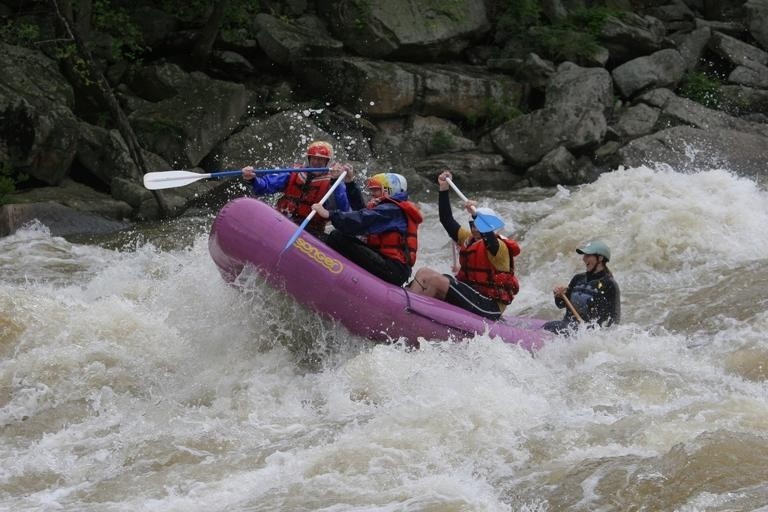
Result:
[207,199,558,357]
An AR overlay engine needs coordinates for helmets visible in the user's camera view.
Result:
[469,208,495,225]
[368,173,407,196]
[308,141,333,160]
[576,242,610,261]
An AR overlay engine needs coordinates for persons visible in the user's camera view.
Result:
[394,174,423,279]
[312,162,408,286]
[242,141,349,235]
[409,171,520,320]
[543,242,621,337]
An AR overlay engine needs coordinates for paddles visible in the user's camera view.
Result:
[143,163,334,191]
[445,175,504,233]
